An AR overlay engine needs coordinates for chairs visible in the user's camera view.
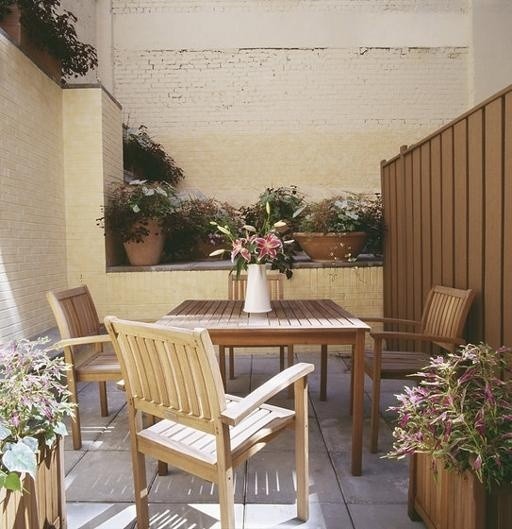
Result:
[349,283,476,451]
[228,273,284,381]
[103,314,316,529]
[46,284,148,450]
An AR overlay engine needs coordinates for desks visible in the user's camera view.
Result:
[153,299,373,477]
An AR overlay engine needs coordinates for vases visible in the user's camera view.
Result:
[243,264,272,315]
[0,427,67,528]
[407,445,512,529]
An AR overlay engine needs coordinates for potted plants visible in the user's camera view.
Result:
[173,198,239,261]
[0,1,98,84]
[294,200,371,261]
[96,180,177,265]
[250,188,303,253]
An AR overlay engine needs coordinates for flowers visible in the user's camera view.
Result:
[379,342,512,494]
[209,201,301,280]
[0,335,80,489]
